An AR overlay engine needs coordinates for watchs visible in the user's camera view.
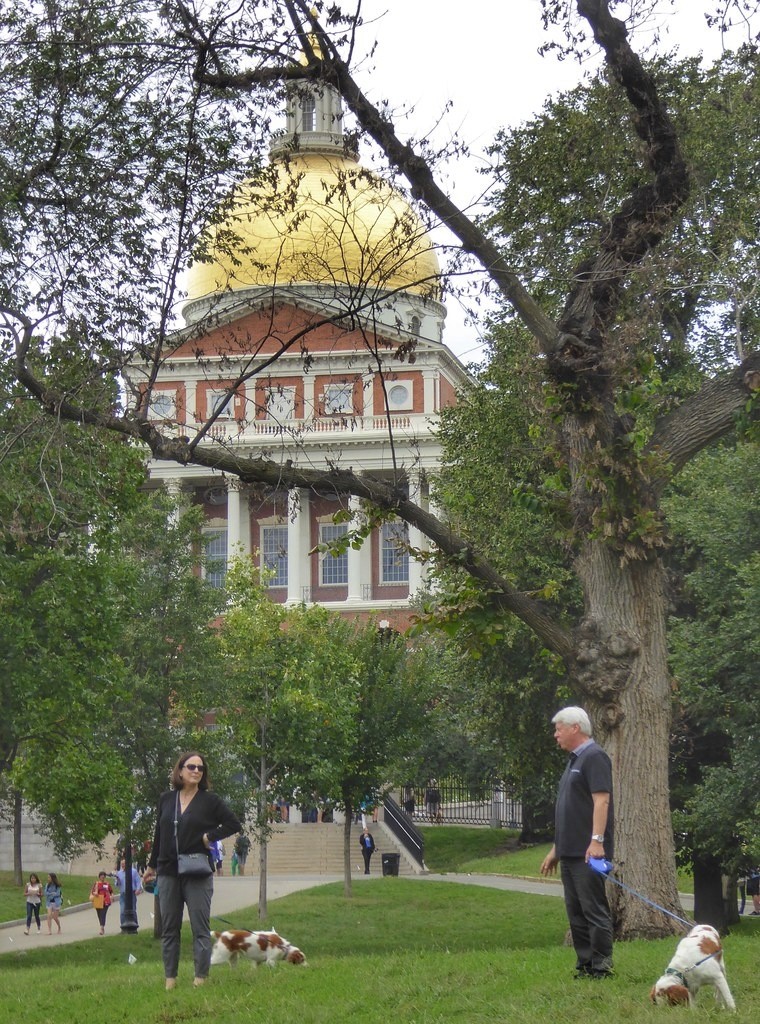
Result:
[592,834,604,842]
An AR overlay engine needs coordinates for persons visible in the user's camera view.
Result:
[209,827,251,876]
[266,772,379,829]
[404,782,415,818]
[90,871,113,935]
[541,706,614,978]
[115,859,143,926]
[143,752,241,988]
[24,873,43,935]
[111,829,152,877]
[45,873,63,935]
[738,865,760,915]
[424,778,443,822]
[359,828,375,874]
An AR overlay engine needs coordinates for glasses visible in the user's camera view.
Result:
[182,764,204,772]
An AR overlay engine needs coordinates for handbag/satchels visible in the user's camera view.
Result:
[177,853,212,878]
[94,895,104,908]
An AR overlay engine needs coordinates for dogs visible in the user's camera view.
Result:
[435,806,444,826]
[210,929,311,969]
[648,924,737,1015]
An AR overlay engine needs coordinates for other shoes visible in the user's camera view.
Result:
[193,980,205,986]
[574,975,592,980]
[165,983,177,991]
[24,929,29,935]
[37,930,40,933]
[100,928,104,935]
[590,975,612,979]
[364,871,370,874]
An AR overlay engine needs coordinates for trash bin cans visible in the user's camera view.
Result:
[382,853,400,877]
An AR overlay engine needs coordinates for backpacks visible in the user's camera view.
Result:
[56,886,63,905]
